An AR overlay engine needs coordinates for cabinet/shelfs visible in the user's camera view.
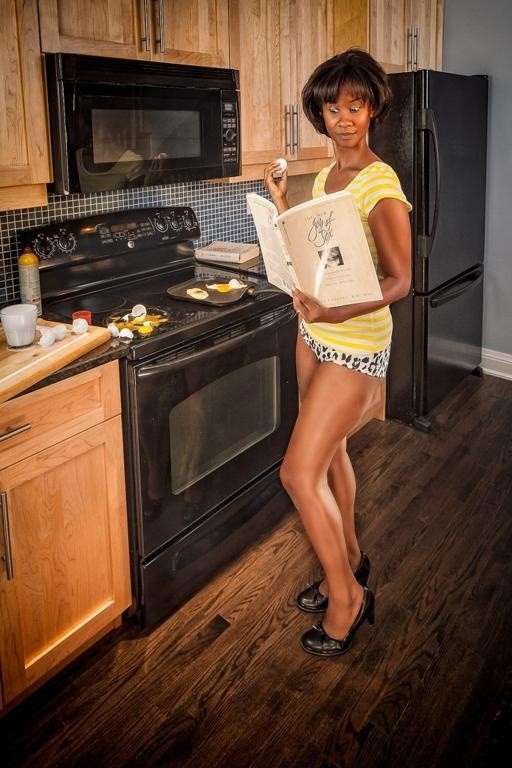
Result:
[0,359,133,715]
[334,0,444,73]
[230,0,333,182]
[38,0,230,68]
[0,0,53,210]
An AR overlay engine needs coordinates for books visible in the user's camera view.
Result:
[245,190,384,308]
[194,241,260,264]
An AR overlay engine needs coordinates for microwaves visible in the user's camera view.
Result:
[41,51,244,200]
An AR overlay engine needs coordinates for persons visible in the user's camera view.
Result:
[75,100,167,194]
[262,50,412,657]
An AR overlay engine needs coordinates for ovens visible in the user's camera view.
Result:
[126,303,300,644]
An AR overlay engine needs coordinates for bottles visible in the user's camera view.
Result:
[17,246,43,318]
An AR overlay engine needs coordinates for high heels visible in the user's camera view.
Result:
[293,554,374,615]
[301,583,379,660]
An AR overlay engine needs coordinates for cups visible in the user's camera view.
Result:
[72,309,92,325]
[0,303,37,347]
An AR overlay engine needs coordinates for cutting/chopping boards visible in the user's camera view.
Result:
[1,318,113,409]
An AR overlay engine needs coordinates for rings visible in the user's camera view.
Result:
[297,311,300,313]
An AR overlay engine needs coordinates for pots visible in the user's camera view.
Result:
[167,272,299,307]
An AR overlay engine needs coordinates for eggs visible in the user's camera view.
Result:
[205,279,247,293]
[187,287,209,300]
[274,158,288,174]
[39,333,55,347]
[72,317,89,334]
[50,325,66,341]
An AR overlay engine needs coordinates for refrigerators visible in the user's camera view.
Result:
[365,68,492,432]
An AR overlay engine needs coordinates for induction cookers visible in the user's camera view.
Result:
[44,266,294,366]
